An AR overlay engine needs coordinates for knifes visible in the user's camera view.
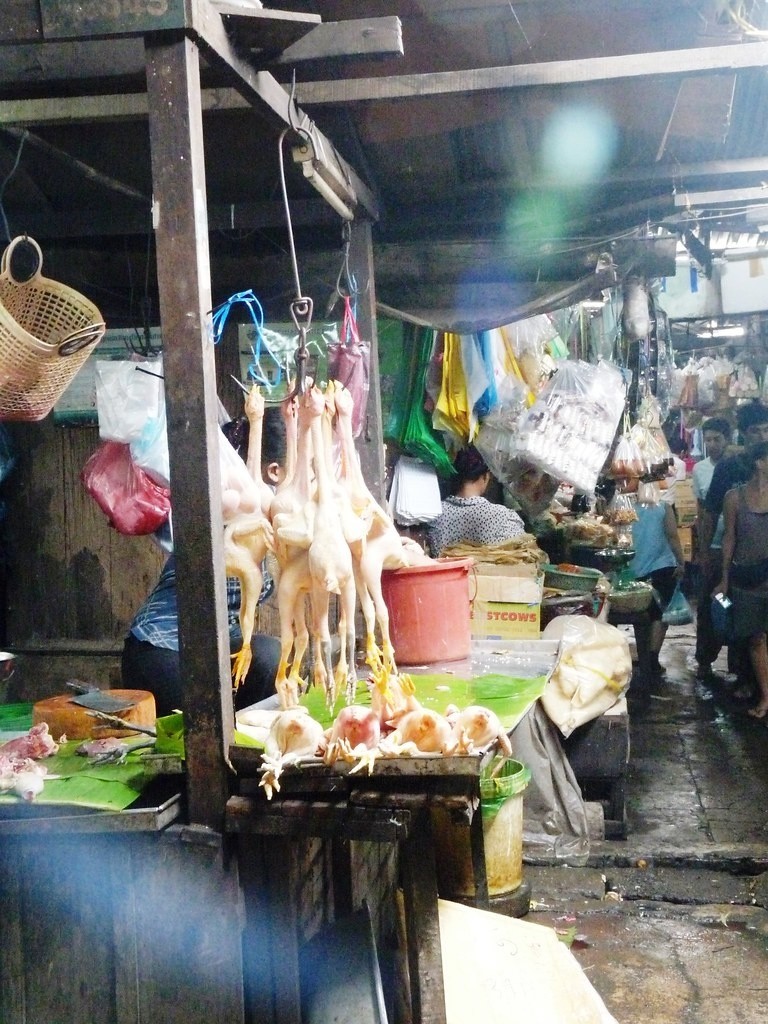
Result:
[65,677,139,713]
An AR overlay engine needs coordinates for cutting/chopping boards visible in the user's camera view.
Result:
[33,689,157,740]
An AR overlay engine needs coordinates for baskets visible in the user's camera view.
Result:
[0,236,107,421]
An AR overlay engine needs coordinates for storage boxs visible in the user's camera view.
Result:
[469,564,545,638]
[672,478,701,563]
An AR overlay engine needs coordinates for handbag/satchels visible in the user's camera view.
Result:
[677,358,759,429]
[730,560,768,586]
[607,389,674,482]
[601,490,640,526]
[327,297,371,440]
[661,576,694,625]
[83,351,257,536]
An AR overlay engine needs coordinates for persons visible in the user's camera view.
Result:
[120,408,298,714]
[632,405,768,717]
[425,448,524,557]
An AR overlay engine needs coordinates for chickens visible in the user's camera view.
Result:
[214,375,512,801]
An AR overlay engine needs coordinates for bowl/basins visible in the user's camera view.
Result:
[0,651,17,680]
[595,549,637,563]
[540,563,603,592]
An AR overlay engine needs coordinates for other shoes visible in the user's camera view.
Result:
[698,664,713,680]
[730,684,756,702]
[657,666,666,673]
[748,706,768,722]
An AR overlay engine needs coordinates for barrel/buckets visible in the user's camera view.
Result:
[380,556,478,666]
